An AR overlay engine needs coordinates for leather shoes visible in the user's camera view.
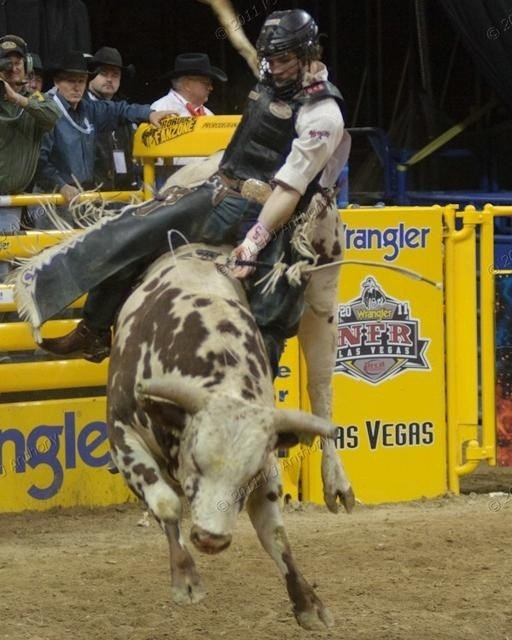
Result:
[36,319,110,363]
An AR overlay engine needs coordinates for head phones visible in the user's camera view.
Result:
[0,35,33,75]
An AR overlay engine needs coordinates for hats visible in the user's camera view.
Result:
[0,34,133,76]
[161,53,229,83]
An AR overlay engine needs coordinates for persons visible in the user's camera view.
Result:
[22,47,181,233]
[2,35,65,273]
[35,10,345,383]
[145,53,228,192]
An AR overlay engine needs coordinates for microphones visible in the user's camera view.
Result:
[16,70,35,86]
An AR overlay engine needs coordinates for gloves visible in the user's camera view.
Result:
[230,221,273,277]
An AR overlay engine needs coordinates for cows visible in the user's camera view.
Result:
[107,149,355,632]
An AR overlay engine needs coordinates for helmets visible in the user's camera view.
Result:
[254,7,320,59]
